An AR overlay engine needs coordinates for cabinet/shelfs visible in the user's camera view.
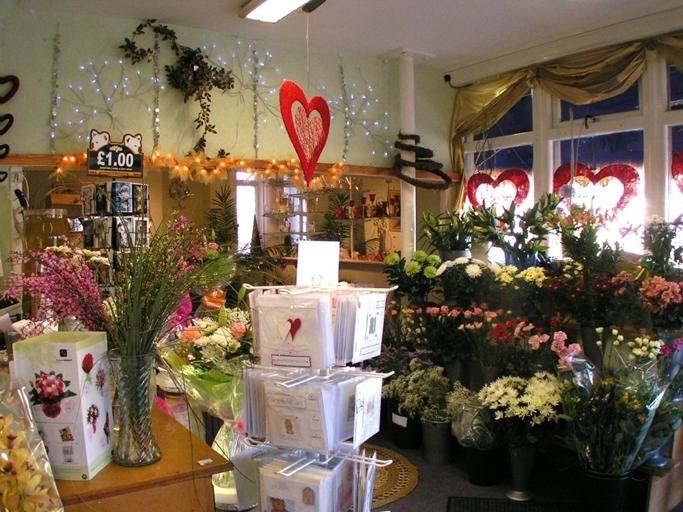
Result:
[14,170,145,310]
[265,166,402,286]
[233,276,400,512]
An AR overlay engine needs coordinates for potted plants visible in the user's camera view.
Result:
[91,182,284,465]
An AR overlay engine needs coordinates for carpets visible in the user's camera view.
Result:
[362,443,420,511]
[446,494,614,511]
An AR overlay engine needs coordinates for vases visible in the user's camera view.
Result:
[506,441,537,500]
[581,471,646,511]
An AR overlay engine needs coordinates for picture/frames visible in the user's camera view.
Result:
[464,452,507,484]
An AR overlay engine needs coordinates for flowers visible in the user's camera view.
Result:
[160,306,258,501]
[4,243,111,335]
